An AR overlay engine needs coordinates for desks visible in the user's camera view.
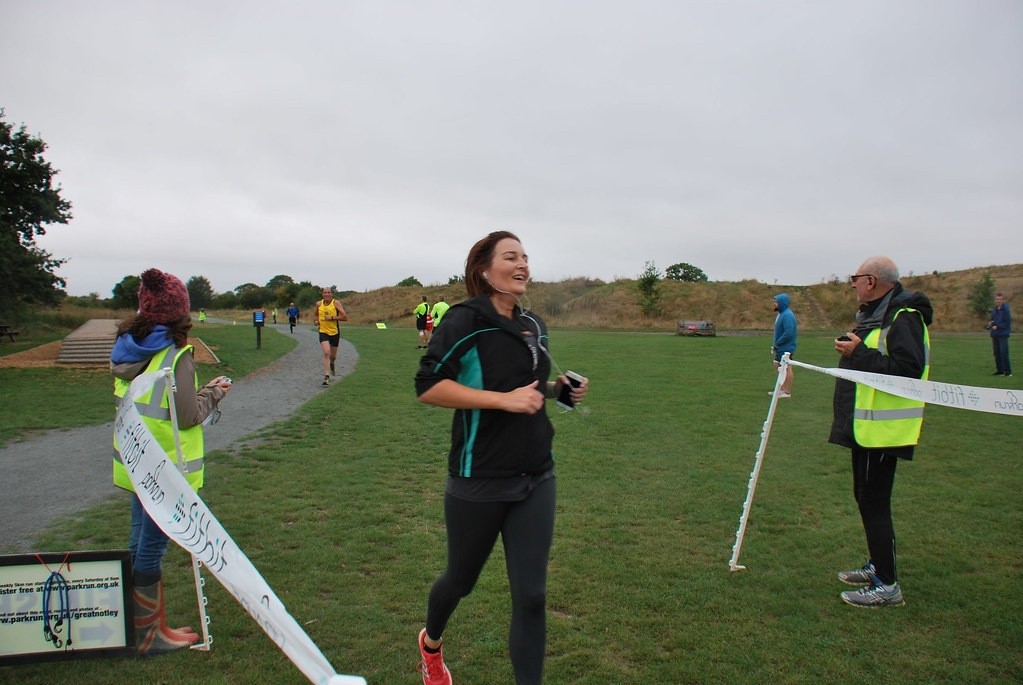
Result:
[0,325,15,342]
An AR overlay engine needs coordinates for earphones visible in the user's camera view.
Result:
[482,272,488,279]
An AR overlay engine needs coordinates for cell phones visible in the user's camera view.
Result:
[556,370,585,411]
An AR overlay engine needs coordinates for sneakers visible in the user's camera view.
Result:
[322,377,331,386]
[837,560,883,587]
[330,364,336,376]
[840,573,905,608]
[415,627,452,685]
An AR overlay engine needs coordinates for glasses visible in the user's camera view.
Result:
[851,274,877,282]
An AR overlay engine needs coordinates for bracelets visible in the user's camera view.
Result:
[332,316,333,320]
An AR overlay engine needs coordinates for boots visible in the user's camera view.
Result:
[124,584,193,650]
[132,578,200,654]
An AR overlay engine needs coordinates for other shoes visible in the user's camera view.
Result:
[1001,372,1013,377]
[778,392,791,399]
[992,371,1005,376]
[422,345,429,349]
[767,389,775,395]
[415,346,423,350]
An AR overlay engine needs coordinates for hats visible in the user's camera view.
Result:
[137,268,190,323]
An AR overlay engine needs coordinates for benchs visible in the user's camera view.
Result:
[0,332,19,334]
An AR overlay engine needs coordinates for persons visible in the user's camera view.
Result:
[427,313,434,342]
[413,295,429,349]
[286,303,300,333]
[768,294,797,397]
[313,287,347,385]
[108,269,231,656]
[272,306,277,324]
[198,308,206,321]
[986,293,1011,376]
[431,294,450,334]
[262,306,266,318]
[828,257,934,608]
[414,231,588,684]
